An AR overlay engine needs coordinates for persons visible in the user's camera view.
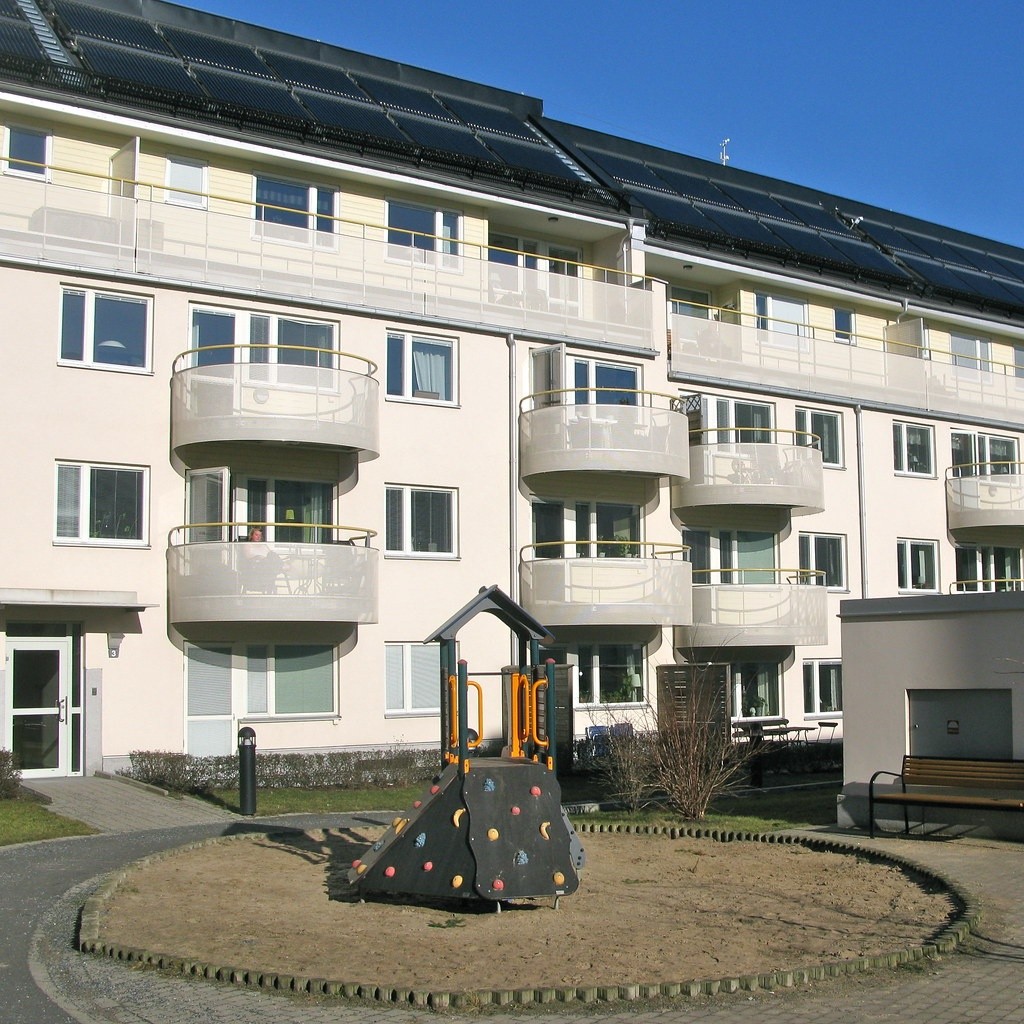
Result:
[750,708,756,716]
[243,527,284,588]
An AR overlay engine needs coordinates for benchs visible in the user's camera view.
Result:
[731,719,790,743]
[869,754,1024,842]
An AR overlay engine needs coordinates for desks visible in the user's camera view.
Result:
[780,726,817,746]
